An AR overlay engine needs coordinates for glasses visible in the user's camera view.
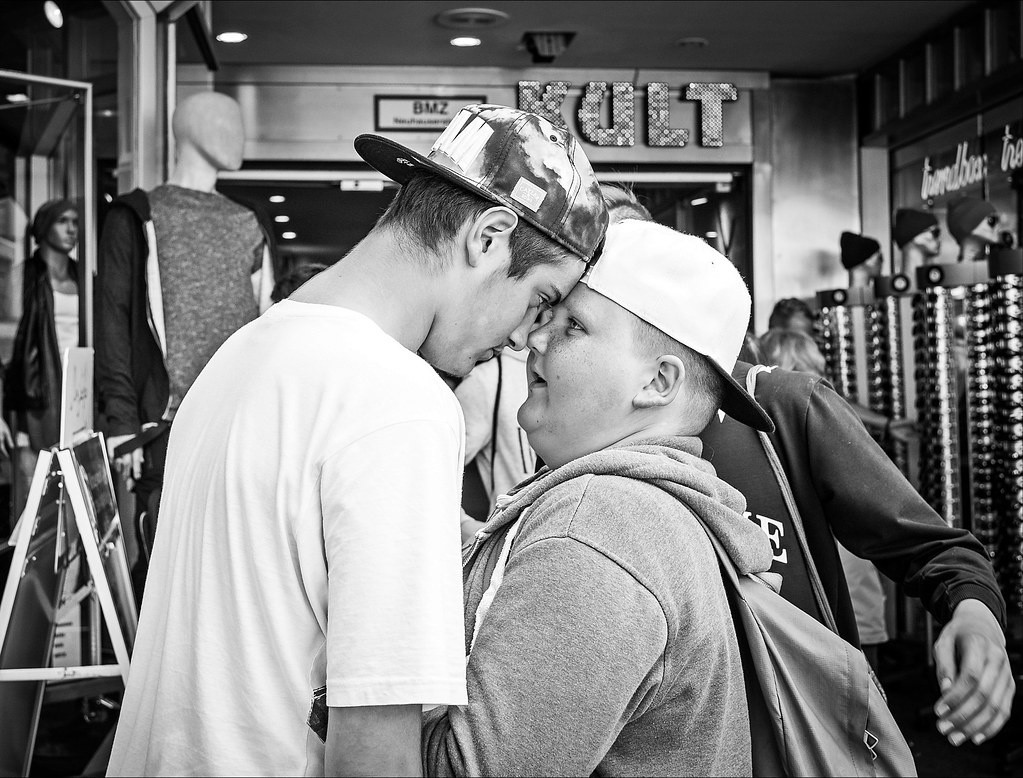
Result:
[924,228,940,239]
[870,255,883,265]
[814,272,1023,638]
[984,215,999,226]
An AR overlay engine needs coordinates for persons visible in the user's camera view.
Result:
[894,209,940,295]
[106,105,610,778]
[454,184,1018,750]
[275,258,329,306]
[839,231,884,303]
[945,195,1003,262]
[2,90,272,479]
[419,223,776,776]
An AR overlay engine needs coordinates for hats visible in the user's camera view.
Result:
[946,194,995,245]
[896,208,937,246]
[579,218,775,433]
[354,104,609,262]
[33,199,78,245]
[841,232,880,269]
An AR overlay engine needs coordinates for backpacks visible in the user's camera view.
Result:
[464,472,920,777]
